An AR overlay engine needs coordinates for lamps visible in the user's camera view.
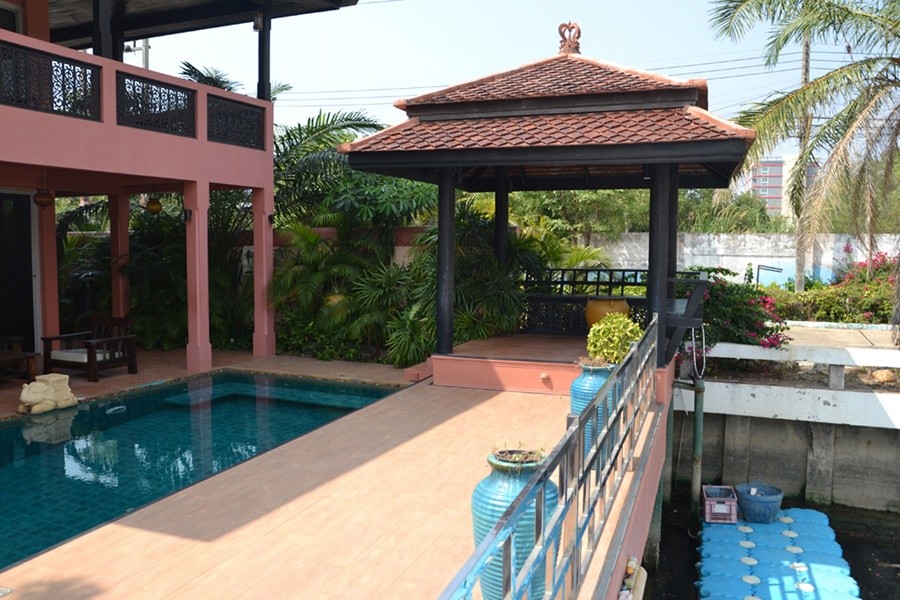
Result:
[147,178,162,214]
[33,168,54,208]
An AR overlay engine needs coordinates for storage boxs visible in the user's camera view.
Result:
[702,485,738,524]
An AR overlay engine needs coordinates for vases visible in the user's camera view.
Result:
[571,357,622,469]
[472,446,563,600]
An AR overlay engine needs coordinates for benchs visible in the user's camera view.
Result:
[527,268,651,333]
[664,270,708,360]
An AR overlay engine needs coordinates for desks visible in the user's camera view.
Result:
[583,294,632,331]
[0,347,41,390]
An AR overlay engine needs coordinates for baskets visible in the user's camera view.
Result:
[736,482,783,524]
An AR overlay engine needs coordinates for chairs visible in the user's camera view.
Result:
[41,315,138,383]
